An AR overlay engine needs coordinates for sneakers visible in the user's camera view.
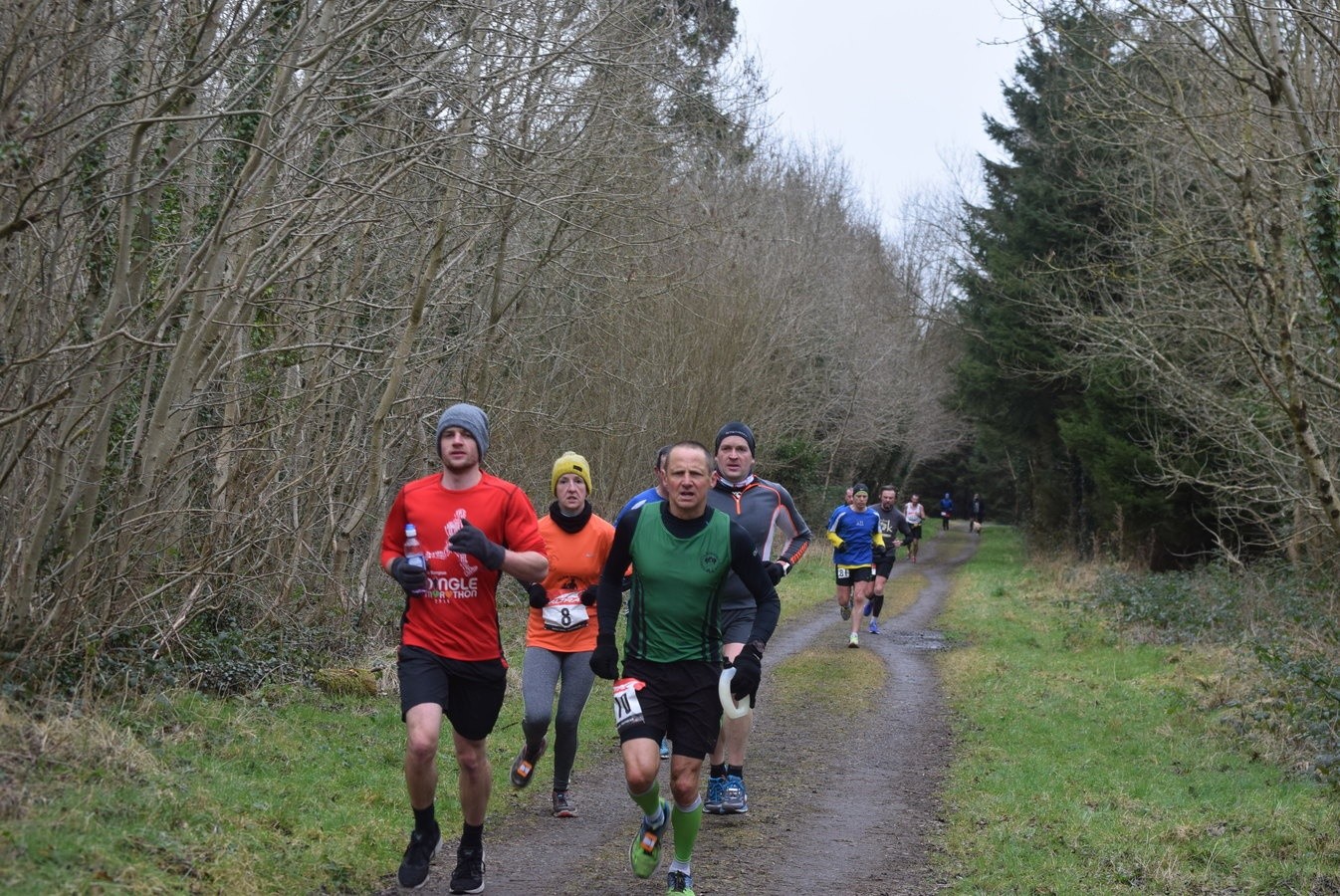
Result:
[449,845,486,894]
[840,594,853,621]
[869,621,877,633]
[665,868,695,896]
[848,635,860,648]
[397,820,443,890]
[510,737,548,789]
[629,798,671,879]
[552,789,579,817]
[863,601,873,617]
[702,764,728,814]
[720,775,749,814]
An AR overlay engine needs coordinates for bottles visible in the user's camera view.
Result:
[404,524,427,593]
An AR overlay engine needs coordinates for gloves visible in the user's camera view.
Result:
[878,545,886,555]
[838,542,846,553]
[581,584,598,606]
[590,634,619,680]
[391,556,430,598]
[762,561,786,587]
[725,645,762,709]
[529,583,550,609]
[446,518,505,572]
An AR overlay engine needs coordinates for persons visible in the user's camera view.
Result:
[939,493,953,531]
[702,422,813,815]
[825,486,854,620]
[864,485,912,635]
[826,483,886,648]
[614,446,668,758]
[589,441,781,896]
[508,451,633,817]
[903,494,927,563]
[379,403,549,894]
[969,492,983,537]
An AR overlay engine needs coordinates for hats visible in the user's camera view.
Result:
[852,483,869,496]
[552,451,592,494]
[435,404,490,463]
[715,422,756,459]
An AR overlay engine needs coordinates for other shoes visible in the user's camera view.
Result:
[907,551,916,563]
[659,735,669,758]
